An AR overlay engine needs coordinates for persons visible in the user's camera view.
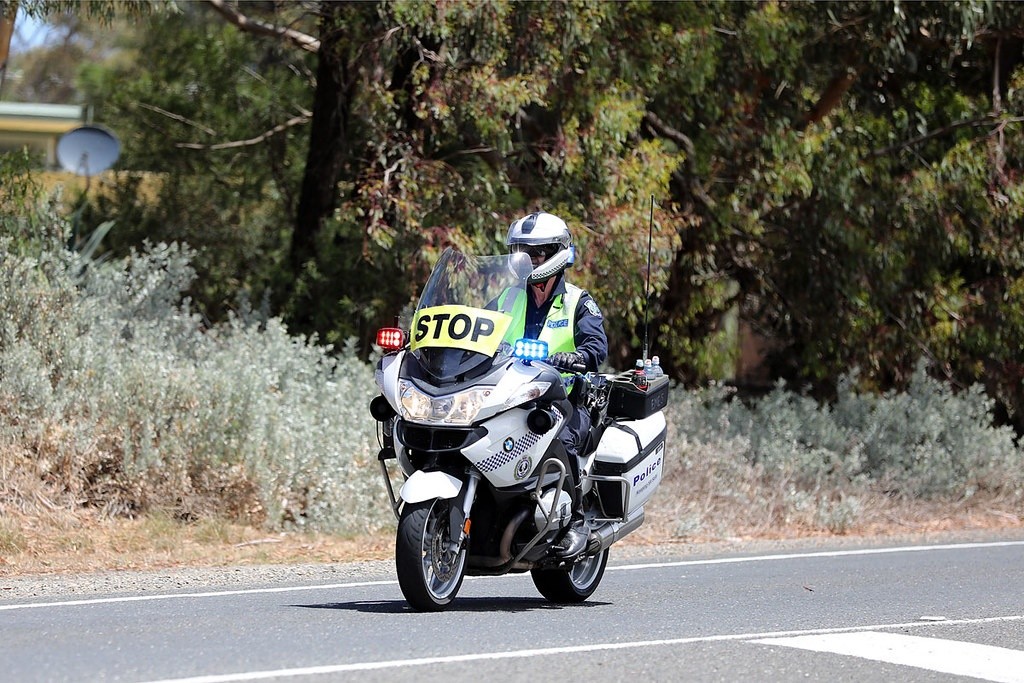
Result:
[480,212,609,556]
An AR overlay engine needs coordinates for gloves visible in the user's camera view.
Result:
[546,351,584,374]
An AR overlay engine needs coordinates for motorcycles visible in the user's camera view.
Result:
[370,249,670,612]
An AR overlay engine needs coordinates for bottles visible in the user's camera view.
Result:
[652,356,663,377]
[632,359,648,391]
[644,359,656,380]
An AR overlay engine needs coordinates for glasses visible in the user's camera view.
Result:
[524,246,551,258]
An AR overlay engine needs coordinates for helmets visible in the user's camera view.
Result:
[504,211,575,284]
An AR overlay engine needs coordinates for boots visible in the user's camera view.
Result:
[555,481,592,558]
